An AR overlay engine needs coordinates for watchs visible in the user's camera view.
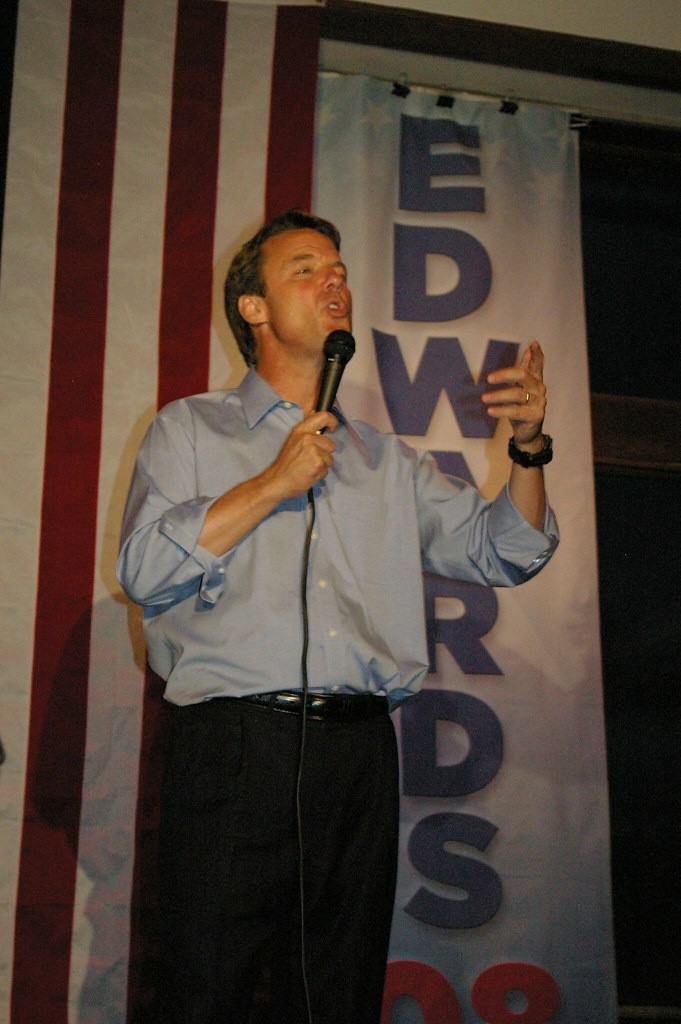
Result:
[508,435,552,467]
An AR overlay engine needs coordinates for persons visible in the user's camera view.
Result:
[119,216,558,1024]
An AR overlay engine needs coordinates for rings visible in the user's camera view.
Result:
[522,391,530,406]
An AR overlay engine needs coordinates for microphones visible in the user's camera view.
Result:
[312,329,357,435]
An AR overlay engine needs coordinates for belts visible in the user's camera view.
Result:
[218,683,389,722]
